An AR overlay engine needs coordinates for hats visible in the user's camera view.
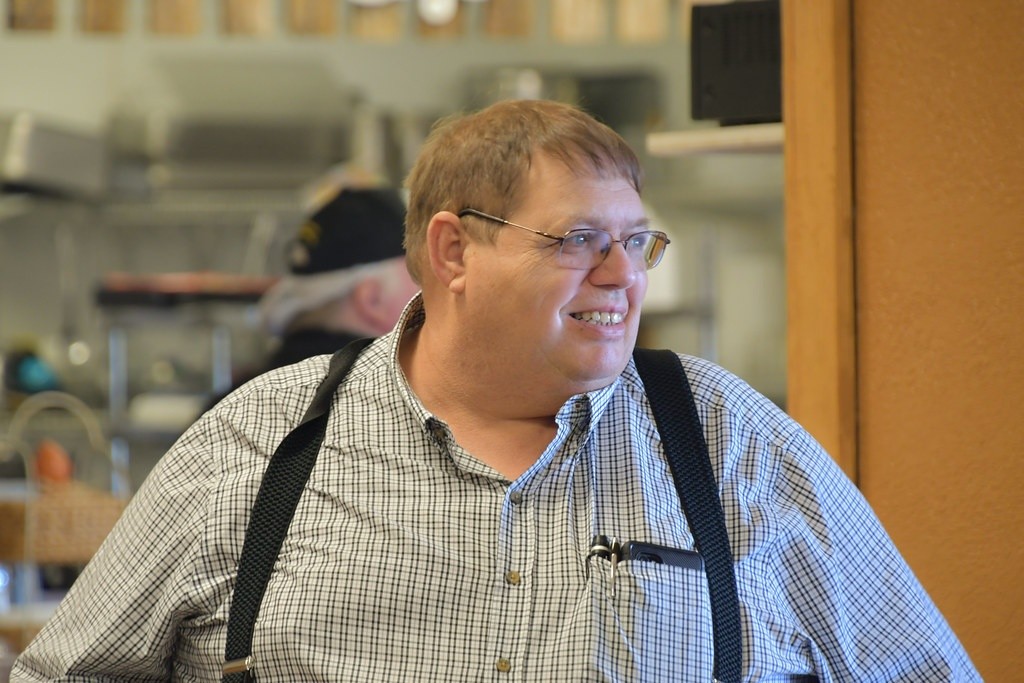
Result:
[289,184,407,274]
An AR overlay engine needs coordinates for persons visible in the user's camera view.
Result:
[8,97,985,683]
[198,183,421,419]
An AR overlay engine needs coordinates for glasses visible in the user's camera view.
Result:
[456,207,669,272]
